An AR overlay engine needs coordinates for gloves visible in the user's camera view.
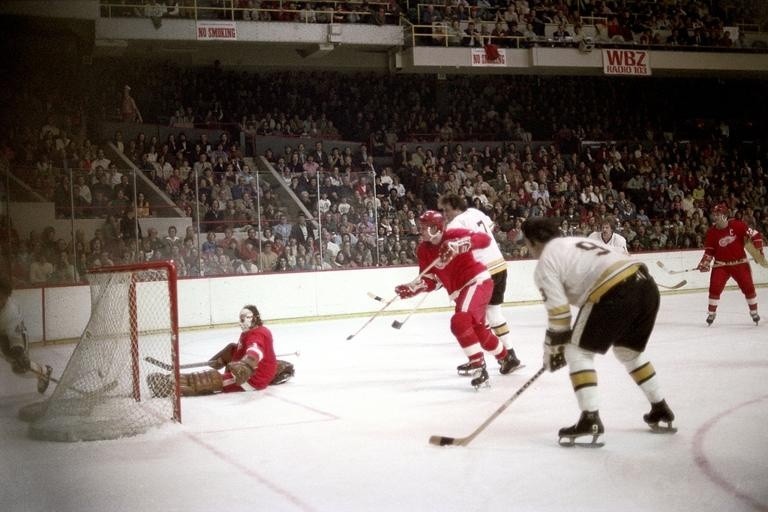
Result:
[543,331,570,371]
[698,253,712,272]
[438,235,471,262]
[394,279,426,298]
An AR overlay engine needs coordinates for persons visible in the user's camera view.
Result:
[585,218,629,258]
[0,1,765,285]
[390,209,520,376]
[520,218,675,438]
[440,193,520,376]
[1,274,51,394]
[207,305,276,392]
[698,205,764,325]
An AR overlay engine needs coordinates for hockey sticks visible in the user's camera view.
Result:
[392,291,429,329]
[347,294,400,341]
[656,280,687,289]
[368,292,390,305]
[30,369,117,397]
[656,258,752,274]
[429,368,546,447]
[144,358,215,370]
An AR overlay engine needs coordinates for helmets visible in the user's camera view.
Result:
[419,210,443,230]
[711,204,729,216]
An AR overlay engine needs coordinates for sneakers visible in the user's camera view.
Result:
[643,399,673,423]
[497,348,518,374]
[558,410,603,437]
[36,365,52,393]
[457,361,488,386]
[706,314,715,323]
[751,313,759,322]
